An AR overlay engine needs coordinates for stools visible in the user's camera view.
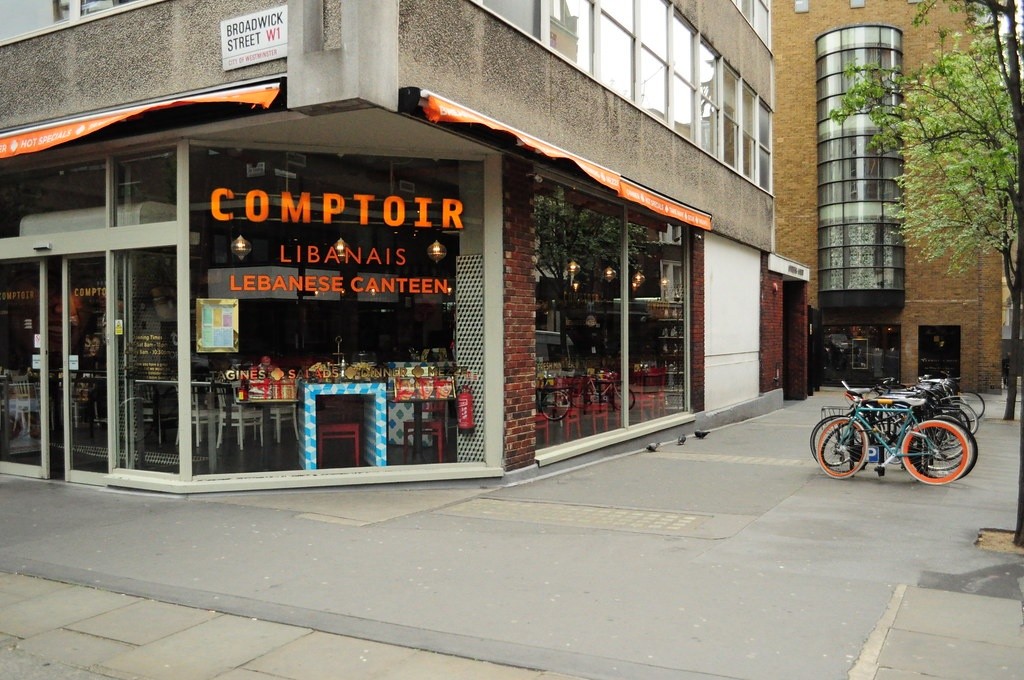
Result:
[318,422,361,468]
[403,418,446,463]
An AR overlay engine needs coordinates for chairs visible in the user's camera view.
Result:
[3,370,101,437]
[171,377,299,451]
[535,367,667,447]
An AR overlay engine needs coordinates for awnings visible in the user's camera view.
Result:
[1,82,280,158]
[399,86,621,193]
[619,175,713,232]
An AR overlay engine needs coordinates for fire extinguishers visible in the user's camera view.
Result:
[456,384,476,432]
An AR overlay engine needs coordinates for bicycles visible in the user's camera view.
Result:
[536,378,571,421]
[569,371,636,411]
[117,376,180,443]
[810,371,986,485]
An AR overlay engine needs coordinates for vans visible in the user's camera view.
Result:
[536,330,583,372]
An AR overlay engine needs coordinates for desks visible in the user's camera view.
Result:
[638,373,662,393]
[247,398,299,471]
[585,380,618,432]
[535,385,574,444]
[387,393,457,463]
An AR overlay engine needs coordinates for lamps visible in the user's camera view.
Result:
[428,240,447,264]
[572,280,581,291]
[566,260,580,275]
[231,235,252,260]
[333,238,351,261]
[632,272,645,286]
[603,268,616,280]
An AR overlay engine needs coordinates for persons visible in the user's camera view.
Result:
[87,337,102,361]
[400,378,452,400]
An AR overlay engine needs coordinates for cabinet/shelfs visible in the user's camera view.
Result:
[659,318,684,413]
